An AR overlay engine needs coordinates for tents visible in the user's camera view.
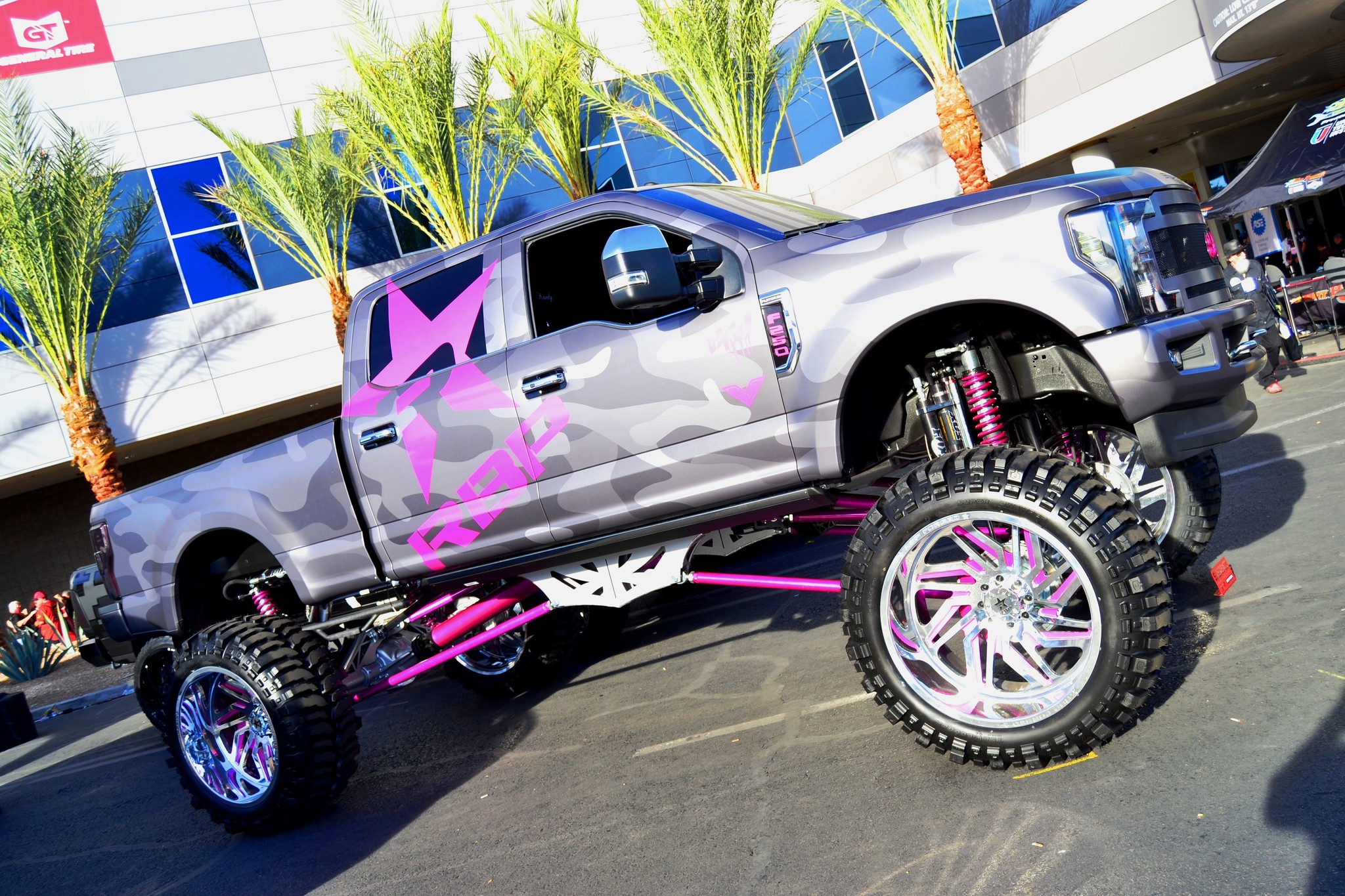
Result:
[1196,91,1345,334]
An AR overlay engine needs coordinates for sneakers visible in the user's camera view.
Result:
[1265,372,1282,393]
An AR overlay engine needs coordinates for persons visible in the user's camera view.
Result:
[1220,238,1286,394]
[1241,213,1345,277]
[6,587,79,649]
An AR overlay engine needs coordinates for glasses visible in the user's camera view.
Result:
[1235,249,1246,257]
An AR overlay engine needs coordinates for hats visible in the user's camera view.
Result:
[33,592,46,599]
[1221,239,1247,259]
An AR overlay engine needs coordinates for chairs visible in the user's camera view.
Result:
[1261,265,1334,342]
[1323,257,1345,350]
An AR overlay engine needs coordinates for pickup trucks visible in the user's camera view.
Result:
[100,107,1269,844]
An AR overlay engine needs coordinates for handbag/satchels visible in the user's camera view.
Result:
[1277,317,1301,362]
